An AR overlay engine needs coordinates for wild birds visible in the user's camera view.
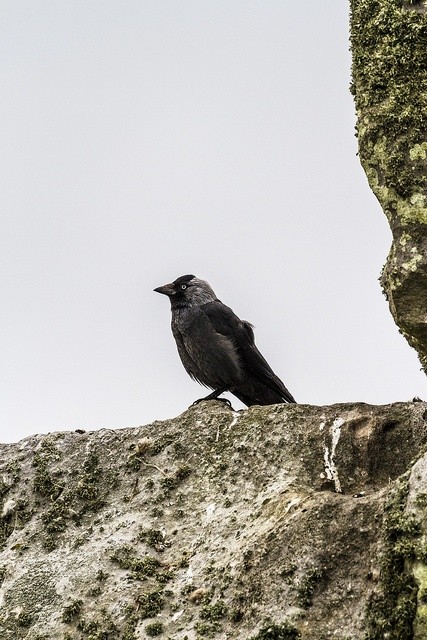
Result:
[153,275,297,410]
